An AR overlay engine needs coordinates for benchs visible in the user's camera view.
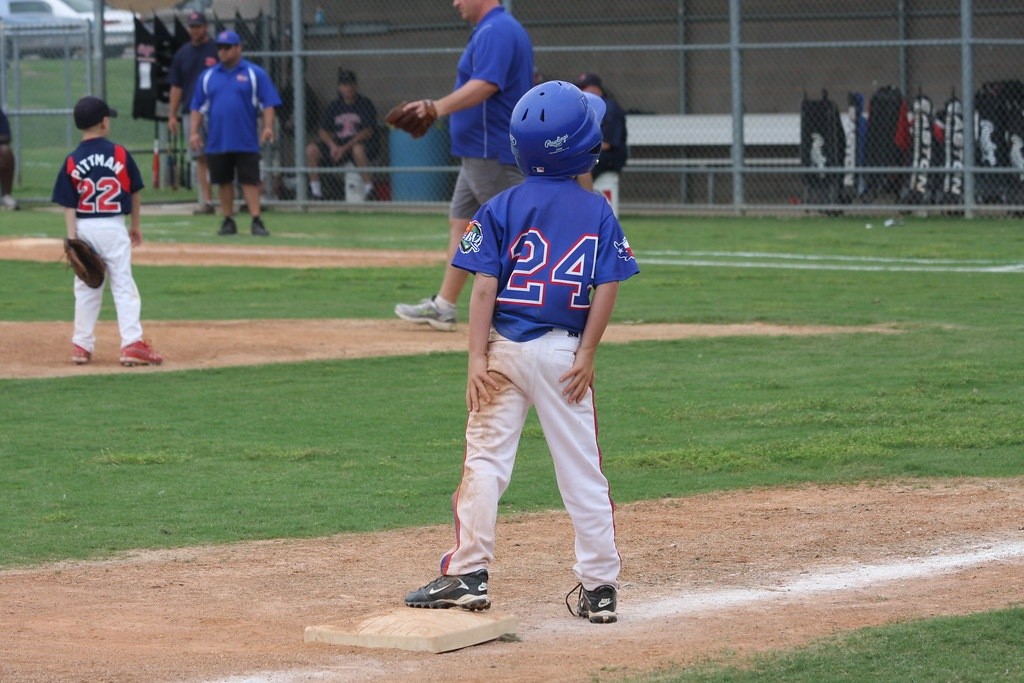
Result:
[626,114,803,207]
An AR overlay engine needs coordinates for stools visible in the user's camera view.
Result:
[594,171,620,222]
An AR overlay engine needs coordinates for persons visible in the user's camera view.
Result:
[168,11,221,216]
[404,77,641,625]
[386,0,534,332]
[50,96,163,367]
[306,70,382,201]
[189,30,284,236]
[0,108,21,211]
[267,70,319,140]
[575,73,626,221]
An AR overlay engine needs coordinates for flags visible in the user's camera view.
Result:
[132,9,274,120]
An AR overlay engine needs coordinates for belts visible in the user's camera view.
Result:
[550,327,579,338]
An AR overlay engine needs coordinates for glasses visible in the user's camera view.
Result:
[216,44,236,50]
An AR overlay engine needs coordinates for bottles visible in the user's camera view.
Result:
[884,219,894,227]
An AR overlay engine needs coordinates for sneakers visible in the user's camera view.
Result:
[395,294,458,332]
[72,342,91,363]
[566,582,617,624]
[218,222,236,235]
[192,204,215,215]
[405,569,492,612]
[252,220,268,235]
[120,339,162,366]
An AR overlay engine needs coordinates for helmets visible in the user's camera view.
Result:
[509,81,607,177]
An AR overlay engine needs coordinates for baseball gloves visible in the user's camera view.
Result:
[58,232,108,290]
[384,95,438,139]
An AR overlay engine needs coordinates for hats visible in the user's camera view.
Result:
[575,73,602,86]
[214,30,240,46]
[74,97,118,128]
[187,12,207,27]
[339,71,356,82]
